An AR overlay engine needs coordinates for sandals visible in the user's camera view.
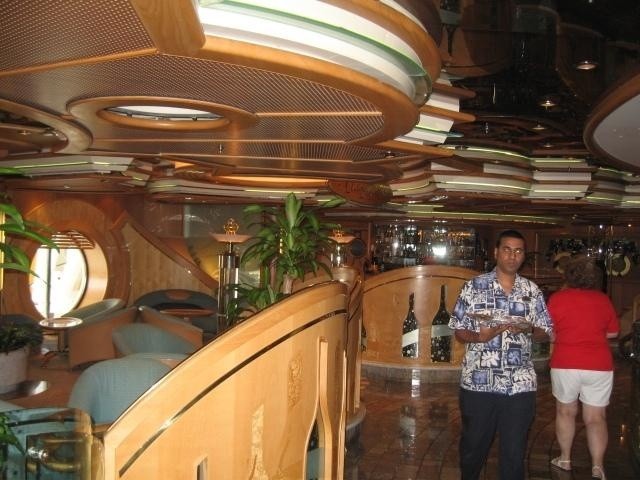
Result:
[592,464,605,480]
[550,456,572,470]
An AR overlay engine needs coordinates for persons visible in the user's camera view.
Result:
[446,228,558,480]
[542,257,622,480]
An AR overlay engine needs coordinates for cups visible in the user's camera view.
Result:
[47,313,55,324]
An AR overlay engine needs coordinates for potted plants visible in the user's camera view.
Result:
[0,168,43,396]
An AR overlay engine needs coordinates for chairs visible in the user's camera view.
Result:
[0,288,219,480]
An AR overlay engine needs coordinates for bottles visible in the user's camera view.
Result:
[402,293,420,360]
[431,284,454,363]
[366,225,475,273]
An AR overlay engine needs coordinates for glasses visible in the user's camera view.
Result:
[500,247,523,254]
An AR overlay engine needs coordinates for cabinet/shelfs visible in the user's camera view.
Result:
[367,221,484,275]
